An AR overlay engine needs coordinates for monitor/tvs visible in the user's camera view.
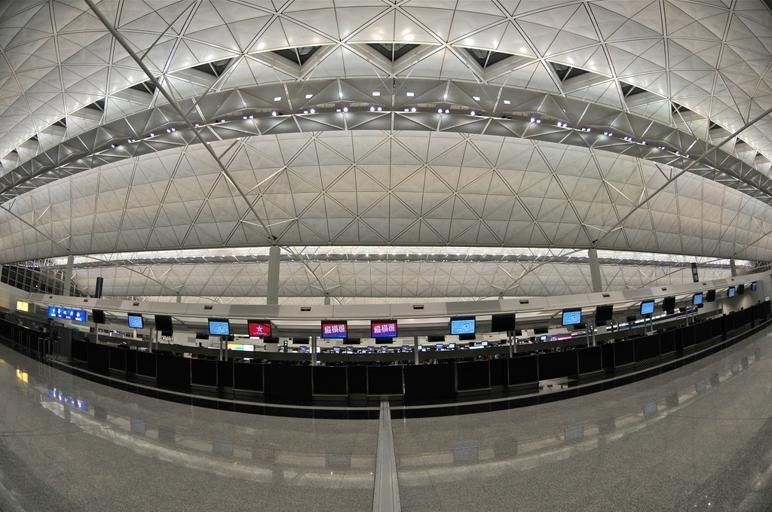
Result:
[155,315,172,331]
[47,306,87,322]
[16,300,29,313]
[664,296,675,309]
[248,320,272,338]
[562,308,582,325]
[321,320,348,338]
[372,320,398,338]
[492,315,515,332]
[640,300,655,315]
[450,316,476,335]
[693,282,757,305]
[208,318,230,336]
[596,306,612,321]
[92,309,105,323]
[298,335,547,354]
[128,313,144,329]
